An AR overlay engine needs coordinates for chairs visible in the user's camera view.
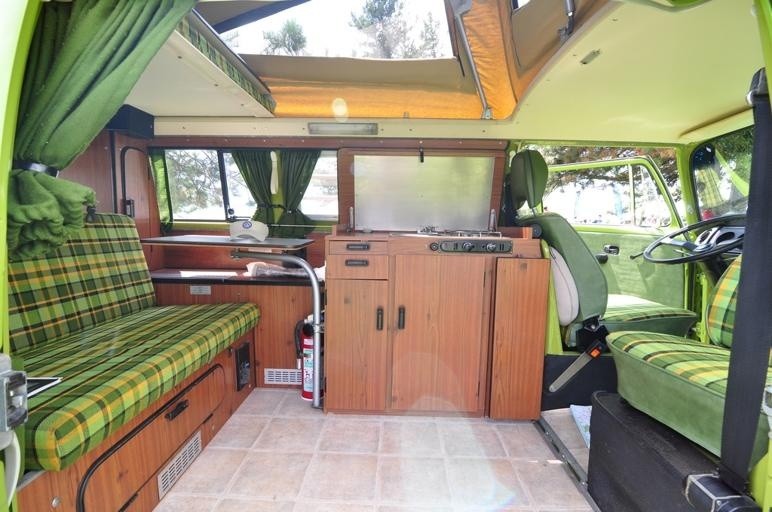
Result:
[508,150,699,349]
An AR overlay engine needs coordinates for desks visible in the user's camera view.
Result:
[139,233,323,408]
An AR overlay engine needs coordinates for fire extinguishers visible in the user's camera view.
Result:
[295,310,325,401]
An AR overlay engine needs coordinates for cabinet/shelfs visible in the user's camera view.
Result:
[324,234,550,422]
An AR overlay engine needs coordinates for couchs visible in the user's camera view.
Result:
[587,251,772,511]
[9,211,260,512]
